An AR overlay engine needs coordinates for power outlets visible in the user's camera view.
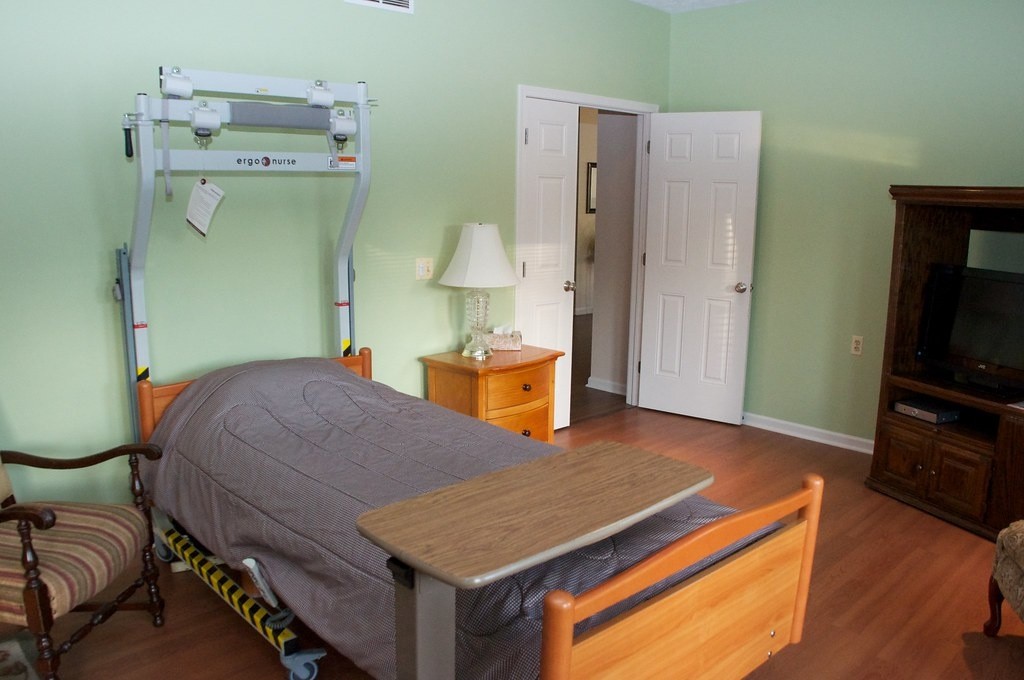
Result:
[850,335,865,355]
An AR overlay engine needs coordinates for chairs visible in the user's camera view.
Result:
[0,440,167,680]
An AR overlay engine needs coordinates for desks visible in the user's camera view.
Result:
[351,437,715,680]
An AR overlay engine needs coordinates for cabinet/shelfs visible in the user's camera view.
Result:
[862,179,1024,545]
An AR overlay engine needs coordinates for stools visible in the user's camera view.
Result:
[980,516,1024,640]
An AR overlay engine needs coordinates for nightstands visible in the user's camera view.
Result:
[422,338,565,444]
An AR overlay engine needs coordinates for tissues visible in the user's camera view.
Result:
[482,324,522,351]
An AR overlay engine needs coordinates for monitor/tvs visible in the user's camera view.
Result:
[914,261,1024,405]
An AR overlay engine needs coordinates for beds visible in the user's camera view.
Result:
[114,347,838,680]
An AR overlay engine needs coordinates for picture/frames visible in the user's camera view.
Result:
[586,161,597,214]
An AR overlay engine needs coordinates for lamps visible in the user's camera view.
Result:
[434,217,521,359]
